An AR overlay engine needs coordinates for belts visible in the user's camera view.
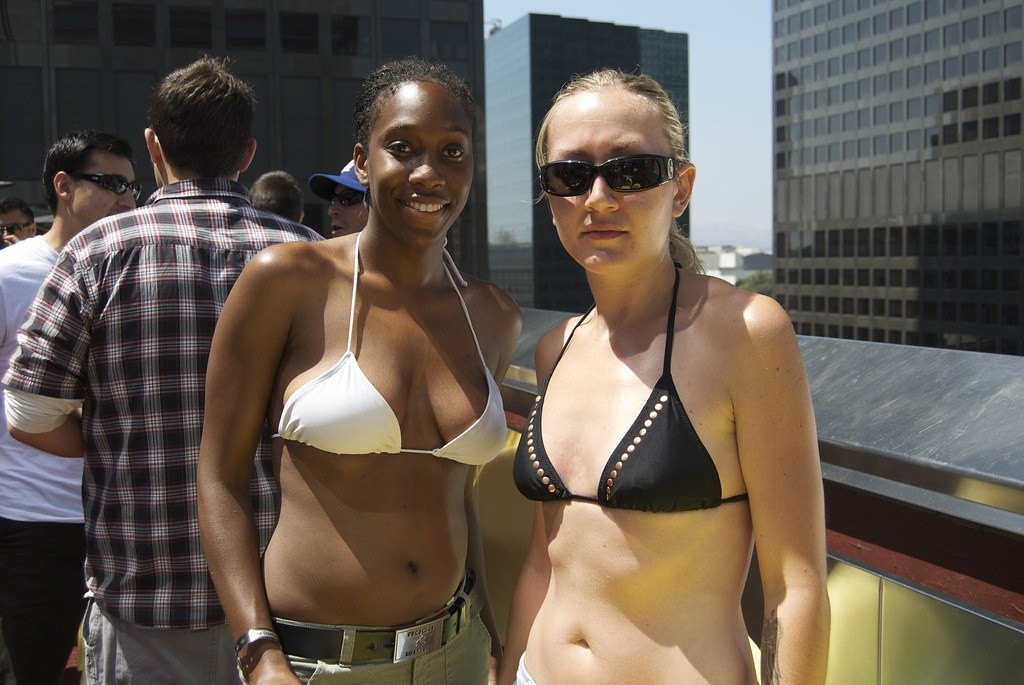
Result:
[264,567,488,668]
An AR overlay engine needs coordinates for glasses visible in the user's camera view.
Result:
[328,190,363,207]
[538,153,680,198]
[0,221,33,233]
[77,171,143,200]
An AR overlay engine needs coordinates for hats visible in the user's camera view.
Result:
[309,158,371,190]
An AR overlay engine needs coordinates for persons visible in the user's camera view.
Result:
[496,67,833,685]
[3,60,334,685]
[1,130,148,684]
[0,154,376,277]
[192,58,525,685]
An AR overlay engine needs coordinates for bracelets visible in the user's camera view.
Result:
[234,627,284,684]
